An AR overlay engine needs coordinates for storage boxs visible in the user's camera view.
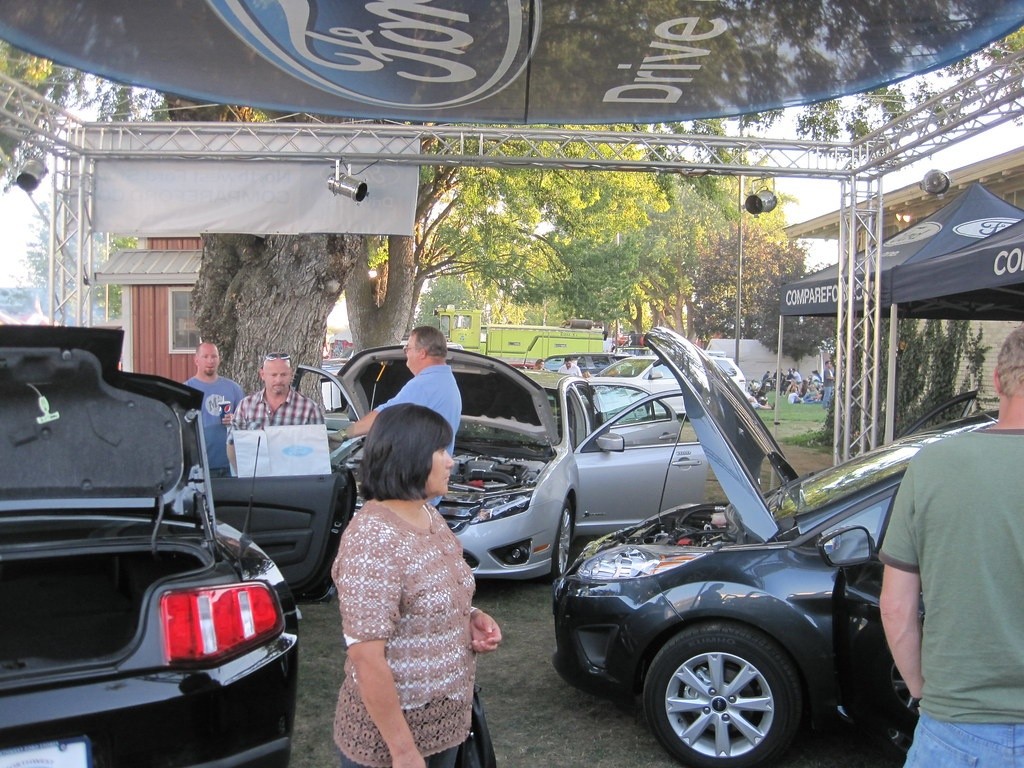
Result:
[225,424,332,478]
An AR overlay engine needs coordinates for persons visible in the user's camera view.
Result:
[330,403,502,768]
[534,357,590,377]
[180,342,245,478]
[328,326,462,511]
[747,361,834,410]
[226,351,325,477]
[879,325,1024,768]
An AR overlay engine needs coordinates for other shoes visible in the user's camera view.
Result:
[771,402,775,410]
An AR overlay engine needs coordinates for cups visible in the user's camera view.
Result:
[217,401,231,425]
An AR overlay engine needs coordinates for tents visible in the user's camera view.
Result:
[767,185,1024,491]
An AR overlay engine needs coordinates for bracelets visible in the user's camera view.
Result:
[338,429,348,440]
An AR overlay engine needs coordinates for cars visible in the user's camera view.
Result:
[577,355,748,421]
[330,344,706,588]
[0,323,358,768]
[542,353,633,375]
[545,322,1008,768]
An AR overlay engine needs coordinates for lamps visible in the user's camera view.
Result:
[918,168,951,196]
[17,158,47,191]
[745,172,778,218]
[327,159,369,207]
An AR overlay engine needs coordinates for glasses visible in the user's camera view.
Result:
[265,353,290,361]
[403,344,418,352]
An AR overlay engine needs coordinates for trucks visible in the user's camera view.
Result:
[434,303,608,361]
